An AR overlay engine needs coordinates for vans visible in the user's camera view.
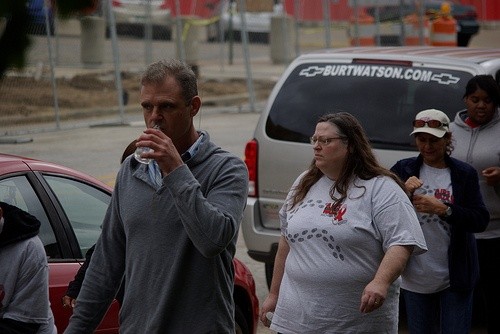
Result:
[241,47,500,290]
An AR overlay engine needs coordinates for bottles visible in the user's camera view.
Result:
[266,311,275,326]
[414,185,430,216]
[134,124,161,165]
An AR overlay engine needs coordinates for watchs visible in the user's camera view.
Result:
[439,207,452,216]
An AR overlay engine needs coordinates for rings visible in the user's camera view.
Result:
[63,304,66,306]
[374,301,379,305]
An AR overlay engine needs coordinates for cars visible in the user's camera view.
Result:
[0,151,260,333]
[106,0,173,39]
[206,0,284,41]
[350,0,481,48]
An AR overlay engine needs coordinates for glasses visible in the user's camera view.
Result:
[310,136,346,145]
[412,119,449,129]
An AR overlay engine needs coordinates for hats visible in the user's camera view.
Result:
[409,108,451,138]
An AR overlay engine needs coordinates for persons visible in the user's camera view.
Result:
[63,58,249,334]
[260,111,427,334]
[0,201,57,334]
[448,74,500,334]
[390,108,491,334]
